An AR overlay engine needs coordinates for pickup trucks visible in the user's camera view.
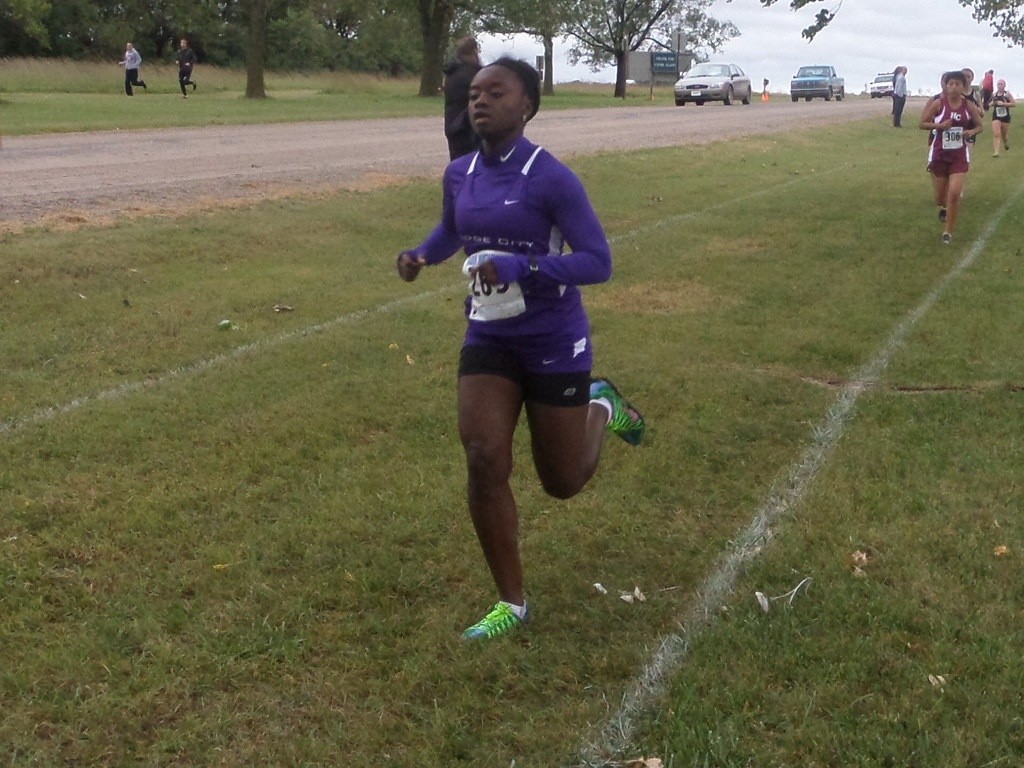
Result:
[790,65,845,101]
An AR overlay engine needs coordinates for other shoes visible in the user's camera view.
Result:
[943,232,951,244]
[937,207,947,222]
[192,81,197,90]
[1004,144,1009,150]
[141,79,146,89]
[992,153,1000,158]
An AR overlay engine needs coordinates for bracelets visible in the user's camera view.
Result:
[525,254,540,279]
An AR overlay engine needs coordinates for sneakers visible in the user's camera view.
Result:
[460,601,529,639]
[590,376,645,444]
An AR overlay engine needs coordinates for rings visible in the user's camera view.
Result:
[482,275,486,280]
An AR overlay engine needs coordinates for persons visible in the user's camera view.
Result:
[396,59,646,644]
[989,81,1016,157]
[891,66,908,127]
[119,41,146,96]
[918,69,993,245]
[175,38,196,98]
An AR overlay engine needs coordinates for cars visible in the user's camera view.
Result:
[870,74,896,98]
[674,62,752,106]
